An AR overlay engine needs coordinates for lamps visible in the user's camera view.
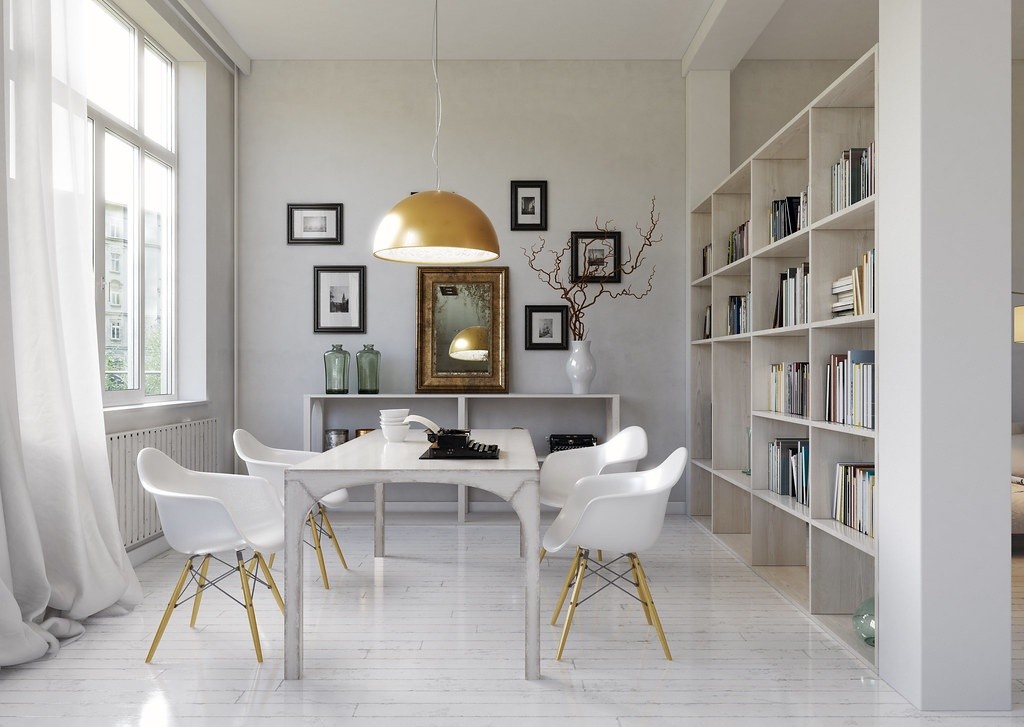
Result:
[372,0,500,266]
[448,326,488,361]
[1013,305,1024,343]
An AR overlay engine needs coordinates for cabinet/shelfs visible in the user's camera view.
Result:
[680,0,1011,715]
[303,393,620,526]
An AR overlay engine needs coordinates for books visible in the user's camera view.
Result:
[771,262,809,328]
[703,305,711,339]
[830,248,875,318]
[726,219,749,265]
[831,461,876,540]
[768,186,809,244]
[824,350,875,430]
[727,291,751,336]
[769,362,809,417]
[702,243,712,277]
[767,438,810,507]
[830,143,877,215]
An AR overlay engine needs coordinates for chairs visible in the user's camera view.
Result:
[542,448,687,661]
[540,425,649,564]
[232,428,348,590]
[136,448,286,664]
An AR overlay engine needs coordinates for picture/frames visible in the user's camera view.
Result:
[525,305,569,350]
[287,203,344,245]
[313,265,367,334]
[510,180,548,232]
[571,231,622,283]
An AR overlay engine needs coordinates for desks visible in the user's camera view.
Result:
[283,429,541,680]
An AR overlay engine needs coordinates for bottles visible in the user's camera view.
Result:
[323,344,350,395]
[852,596,875,648]
[356,344,382,394]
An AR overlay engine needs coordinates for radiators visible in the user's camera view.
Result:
[106,416,220,553]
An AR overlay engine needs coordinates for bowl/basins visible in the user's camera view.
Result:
[379,408,411,443]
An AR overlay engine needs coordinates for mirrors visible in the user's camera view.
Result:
[415,265,509,393]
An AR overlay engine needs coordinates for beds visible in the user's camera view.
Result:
[1011,421,1024,550]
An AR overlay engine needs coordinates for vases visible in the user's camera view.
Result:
[323,343,351,395]
[355,344,382,395]
[567,341,596,395]
